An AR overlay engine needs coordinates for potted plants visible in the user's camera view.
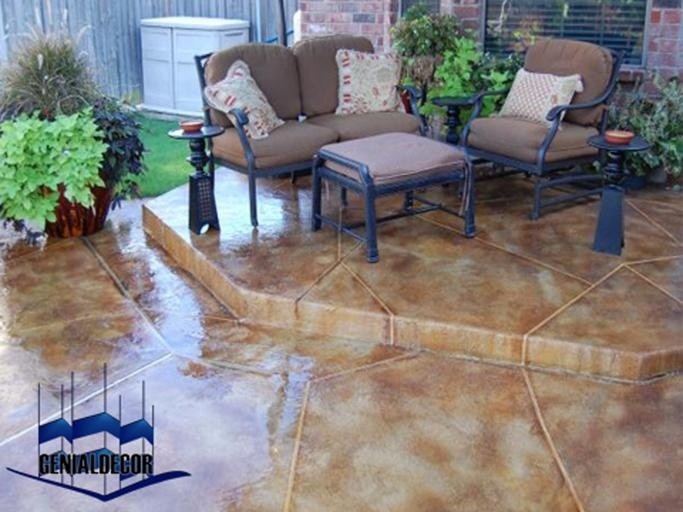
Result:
[0,0,153,248]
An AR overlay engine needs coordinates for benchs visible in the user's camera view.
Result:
[194,32,427,225]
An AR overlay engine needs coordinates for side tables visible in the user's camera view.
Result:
[169,125,226,235]
[432,96,473,189]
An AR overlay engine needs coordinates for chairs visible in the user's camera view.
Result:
[430,38,625,221]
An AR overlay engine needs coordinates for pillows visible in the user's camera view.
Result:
[204,58,286,140]
[497,65,585,132]
[334,46,407,117]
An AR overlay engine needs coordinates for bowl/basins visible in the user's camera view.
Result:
[180,120,203,132]
[604,130,635,143]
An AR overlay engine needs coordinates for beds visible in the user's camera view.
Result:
[585,135,650,256]
